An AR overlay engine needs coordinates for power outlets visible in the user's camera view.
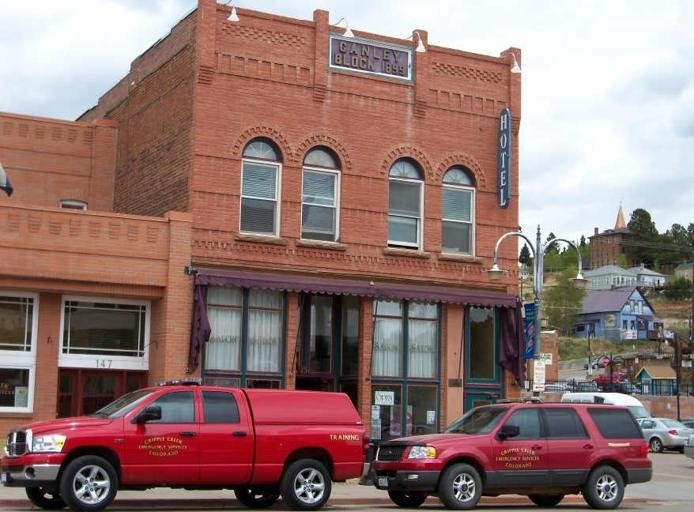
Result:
[0,380,369,511]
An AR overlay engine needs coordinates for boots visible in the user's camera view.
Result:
[358,401,653,507]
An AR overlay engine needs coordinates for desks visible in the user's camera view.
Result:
[484,223,588,392]
[596,353,626,390]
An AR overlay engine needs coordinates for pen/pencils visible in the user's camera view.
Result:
[561,391,650,418]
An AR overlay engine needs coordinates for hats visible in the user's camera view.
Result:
[632,419,694,453]
[543,369,642,393]
[584,357,606,369]
